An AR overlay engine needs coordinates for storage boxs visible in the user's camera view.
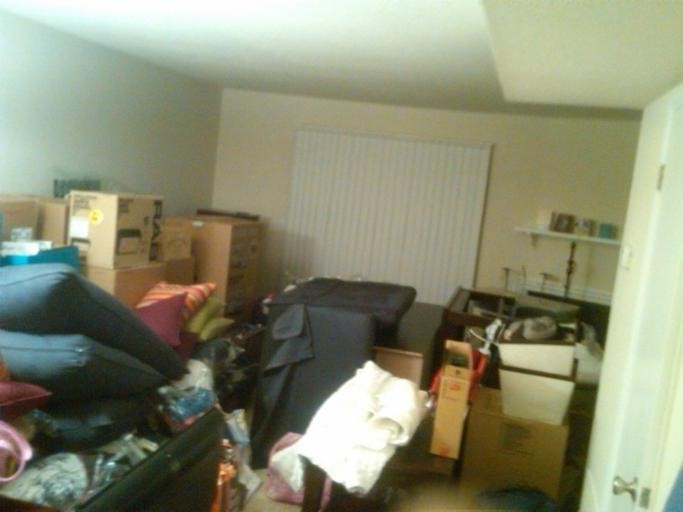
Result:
[429,317,581,512]
[0,190,265,323]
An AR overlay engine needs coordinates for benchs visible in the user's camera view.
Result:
[372,300,446,390]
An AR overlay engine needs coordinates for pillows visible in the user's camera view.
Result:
[1,382,50,423]
[135,279,218,329]
[37,397,159,452]
[3,416,35,457]
[0,326,175,393]
[184,296,228,334]
[200,316,233,341]
[133,289,189,348]
[1,262,193,382]
